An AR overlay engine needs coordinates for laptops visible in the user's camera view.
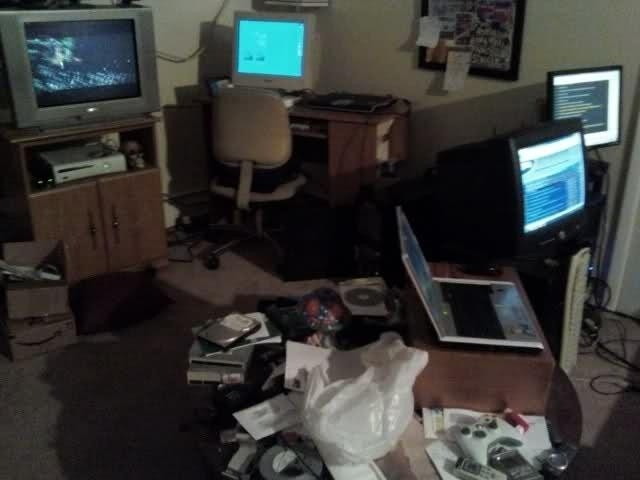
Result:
[395,206,545,353]
[311,93,391,113]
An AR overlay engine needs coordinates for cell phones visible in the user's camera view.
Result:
[491,447,544,480]
[542,442,577,477]
[453,457,507,480]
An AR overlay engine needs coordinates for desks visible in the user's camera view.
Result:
[205,92,410,240]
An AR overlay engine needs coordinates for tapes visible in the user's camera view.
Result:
[258,440,323,480]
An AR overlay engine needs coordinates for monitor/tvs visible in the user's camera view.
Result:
[0,8,160,131]
[232,11,320,92]
[547,65,622,177]
[434,117,592,257]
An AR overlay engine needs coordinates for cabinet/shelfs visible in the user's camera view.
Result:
[3,117,173,284]
[393,260,554,422]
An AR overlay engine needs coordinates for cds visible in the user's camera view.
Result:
[345,288,384,306]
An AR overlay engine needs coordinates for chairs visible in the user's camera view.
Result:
[196,87,318,272]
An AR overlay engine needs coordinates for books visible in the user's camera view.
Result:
[192,312,283,358]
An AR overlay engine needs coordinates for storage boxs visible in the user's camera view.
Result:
[0,238,81,362]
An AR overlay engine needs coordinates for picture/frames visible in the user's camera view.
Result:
[414,2,528,82]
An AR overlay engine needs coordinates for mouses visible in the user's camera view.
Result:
[462,261,503,277]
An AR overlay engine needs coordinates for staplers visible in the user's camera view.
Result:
[220,441,265,480]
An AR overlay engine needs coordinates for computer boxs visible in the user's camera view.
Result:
[526,245,591,379]
